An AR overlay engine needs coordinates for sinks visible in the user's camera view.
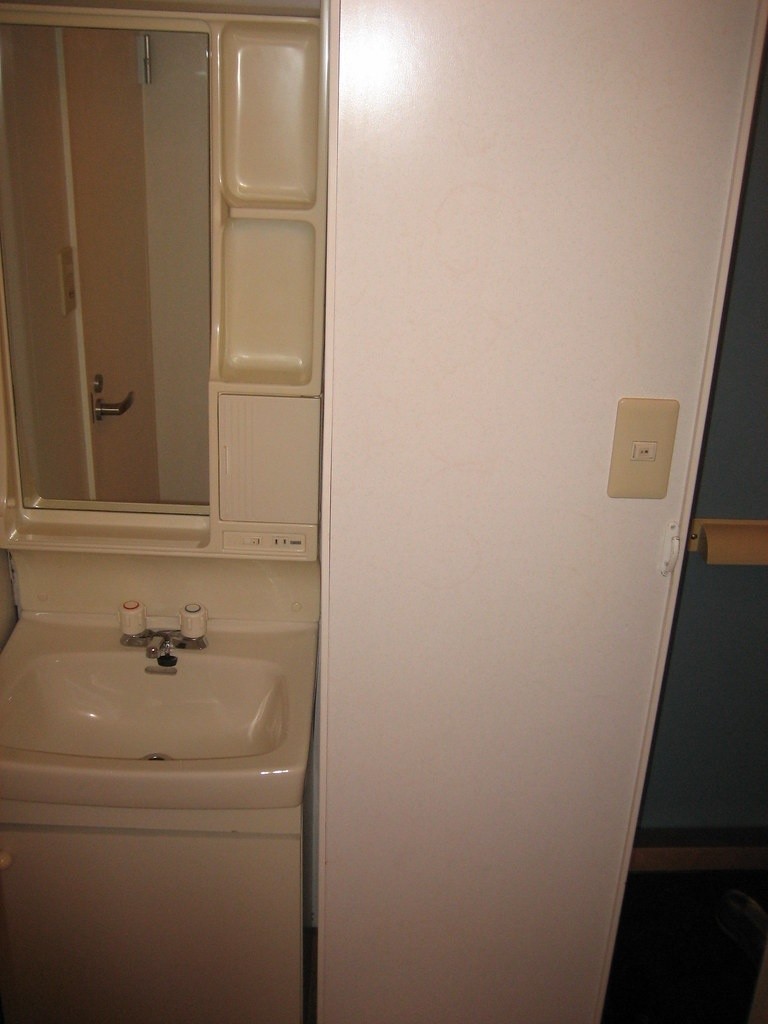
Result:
[0,618,316,808]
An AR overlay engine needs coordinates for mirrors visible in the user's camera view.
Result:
[0,11,213,515]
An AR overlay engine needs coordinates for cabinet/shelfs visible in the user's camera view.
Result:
[0,799,304,1024]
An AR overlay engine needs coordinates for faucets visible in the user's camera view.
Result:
[146,636,164,659]
